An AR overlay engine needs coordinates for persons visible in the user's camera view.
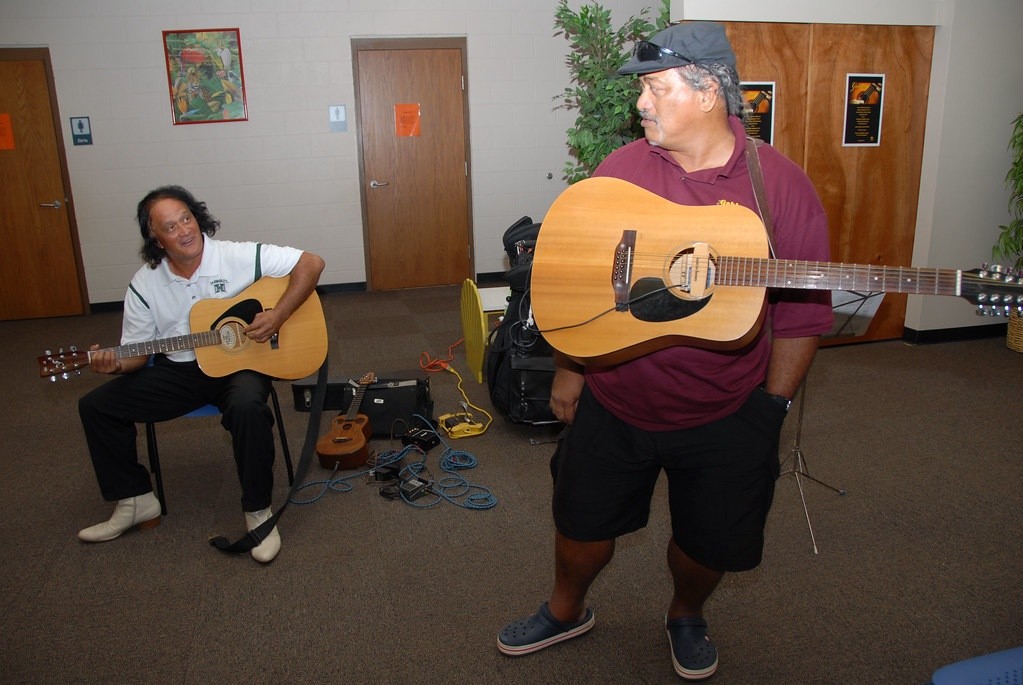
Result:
[497,19,837,679]
[78,184,325,565]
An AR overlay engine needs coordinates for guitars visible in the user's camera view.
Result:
[39,275,329,382]
[316,371,378,468]
[527,176,1022,372]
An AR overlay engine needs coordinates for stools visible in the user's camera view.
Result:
[460,278,515,383]
[140,349,294,517]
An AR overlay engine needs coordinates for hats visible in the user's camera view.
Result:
[617,23,736,75]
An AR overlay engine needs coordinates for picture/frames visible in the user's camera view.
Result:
[161,27,249,126]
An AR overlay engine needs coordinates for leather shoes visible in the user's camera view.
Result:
[77,491,162,543]
[244,504,282,563]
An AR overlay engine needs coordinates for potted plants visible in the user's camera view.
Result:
[991,112,1023,353]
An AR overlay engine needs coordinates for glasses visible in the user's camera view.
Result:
[633,41,696,64]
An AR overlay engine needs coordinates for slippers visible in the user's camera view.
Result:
[496,600,596,656]
[664,609,719,679]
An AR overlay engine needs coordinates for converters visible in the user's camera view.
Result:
[383,465,400,477]
[374,468,391,481]
[398,480,432,503]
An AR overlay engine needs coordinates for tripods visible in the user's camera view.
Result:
[778,376,847,558]
